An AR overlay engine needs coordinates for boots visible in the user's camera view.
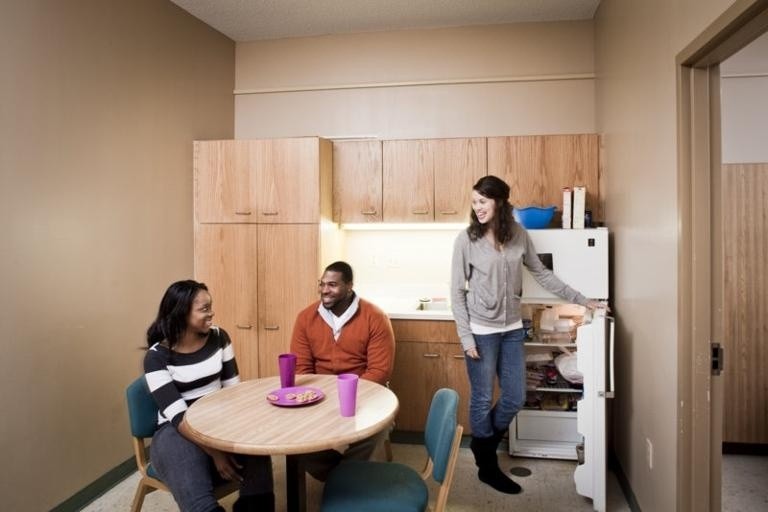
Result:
[469,428,522,496]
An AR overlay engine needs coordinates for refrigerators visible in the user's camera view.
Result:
[507,227,616,511]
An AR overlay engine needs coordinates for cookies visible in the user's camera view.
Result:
[267,394,278,401]
[287,391,317,402]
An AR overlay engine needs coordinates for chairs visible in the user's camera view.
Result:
[385,381,393,462]
[125,373,189,512]
[322,387,463,512]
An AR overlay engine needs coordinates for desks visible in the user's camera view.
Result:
[183,373,399,510]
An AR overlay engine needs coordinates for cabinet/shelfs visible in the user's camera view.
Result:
[193,139,333,224]
[334,133,599,224]
[386,321,502,436]
[193,224,333,383]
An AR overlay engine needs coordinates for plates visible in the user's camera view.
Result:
[266,386,324,407]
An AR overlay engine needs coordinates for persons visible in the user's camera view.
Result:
[289,261,395,484]
[452,176,612,492]
[143,280,274,512]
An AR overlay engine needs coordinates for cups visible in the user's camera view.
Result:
[337,374,358,417]
[279,354,296,388]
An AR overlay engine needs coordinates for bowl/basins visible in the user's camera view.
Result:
[513,206,558,228]
